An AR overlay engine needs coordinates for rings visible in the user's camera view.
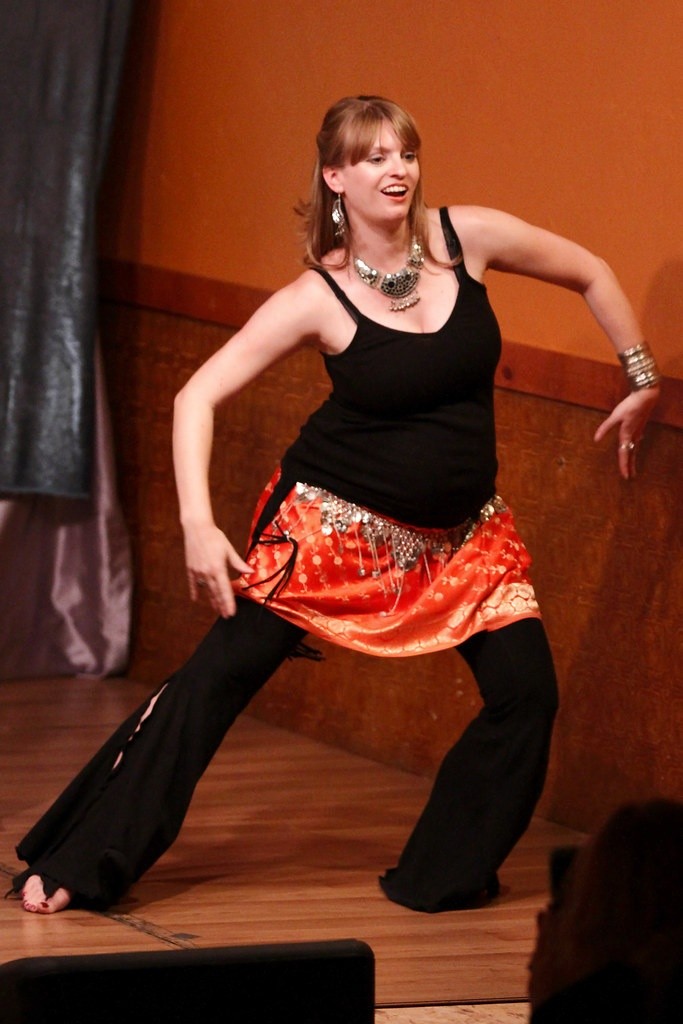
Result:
[620,441,635,450]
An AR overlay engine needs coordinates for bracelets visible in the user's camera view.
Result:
[617,341,661,388]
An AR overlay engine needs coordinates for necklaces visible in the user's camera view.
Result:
[346,235,426,313]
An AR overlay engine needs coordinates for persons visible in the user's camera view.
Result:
[527,799,683,1024]
[11,95,662,915]
